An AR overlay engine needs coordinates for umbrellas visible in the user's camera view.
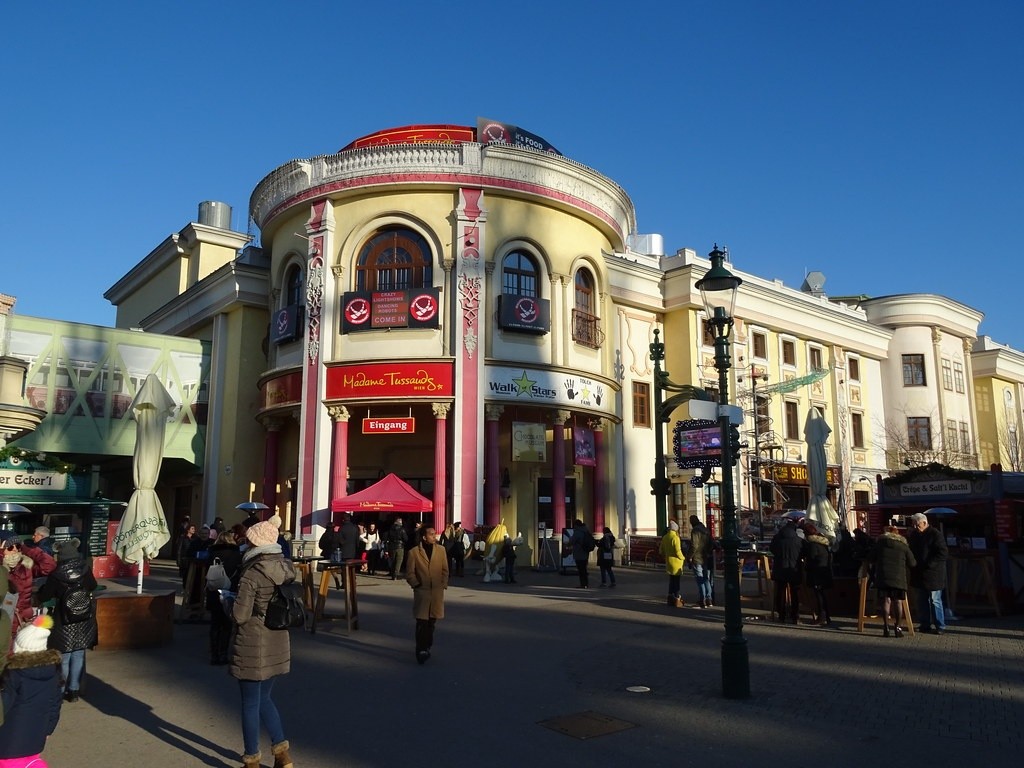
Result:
[803,407,840,538]
[110,374,177,594]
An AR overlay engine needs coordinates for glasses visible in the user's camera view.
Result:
[5,543,22,552]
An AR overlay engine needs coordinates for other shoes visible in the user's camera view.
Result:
[66,687,80,702]
[599,584,606,588]
[697,600,713,608]
[417,651,431,665]
[609,583,616,587]
[937,628,945,635]
[915,625,932,632]
[812,616,830,625]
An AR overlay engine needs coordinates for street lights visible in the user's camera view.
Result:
[694,242,751,699]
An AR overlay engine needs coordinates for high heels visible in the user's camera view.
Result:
[894,624,905,638]
[884,625,890,636]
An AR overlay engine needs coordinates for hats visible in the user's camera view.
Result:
[670,520,679,532]
[57,538,80,558]
[245,515,281,546]
[14,614,54,656]
[0,529,24,549]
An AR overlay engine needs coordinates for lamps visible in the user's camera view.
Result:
[235,502,269,518]
[923,508,959,535]
[781,511,806,524]
[0,503,32,523]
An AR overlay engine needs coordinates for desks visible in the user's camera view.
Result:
[291,553,368,633]
[737,550,1001,635]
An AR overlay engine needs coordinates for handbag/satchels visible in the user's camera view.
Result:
[603,553,612,560]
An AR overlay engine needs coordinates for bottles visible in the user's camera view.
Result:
[298,546,304,558]
[334,548,342,562]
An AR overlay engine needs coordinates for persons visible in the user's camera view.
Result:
[319,518,464,580]
[570,520,589,589]
[658,520,686,607]
[688,515,714,608]
[177,515,296,768]
[769,513,952,638]
[502,539,517,584]
[405,524,449,666]
[0,521,98,768]
[595,527,616,588]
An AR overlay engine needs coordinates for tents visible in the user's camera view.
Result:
[330,473,433,522]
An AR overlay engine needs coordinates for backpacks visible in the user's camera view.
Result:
[206,557,237,591]
[60,580,93,624]
[255,577,307,630]
[577,531,595,552]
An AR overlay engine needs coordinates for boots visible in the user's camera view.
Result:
[271,739,293,768]
[240,750,261,768]
[667,594,683,607]
